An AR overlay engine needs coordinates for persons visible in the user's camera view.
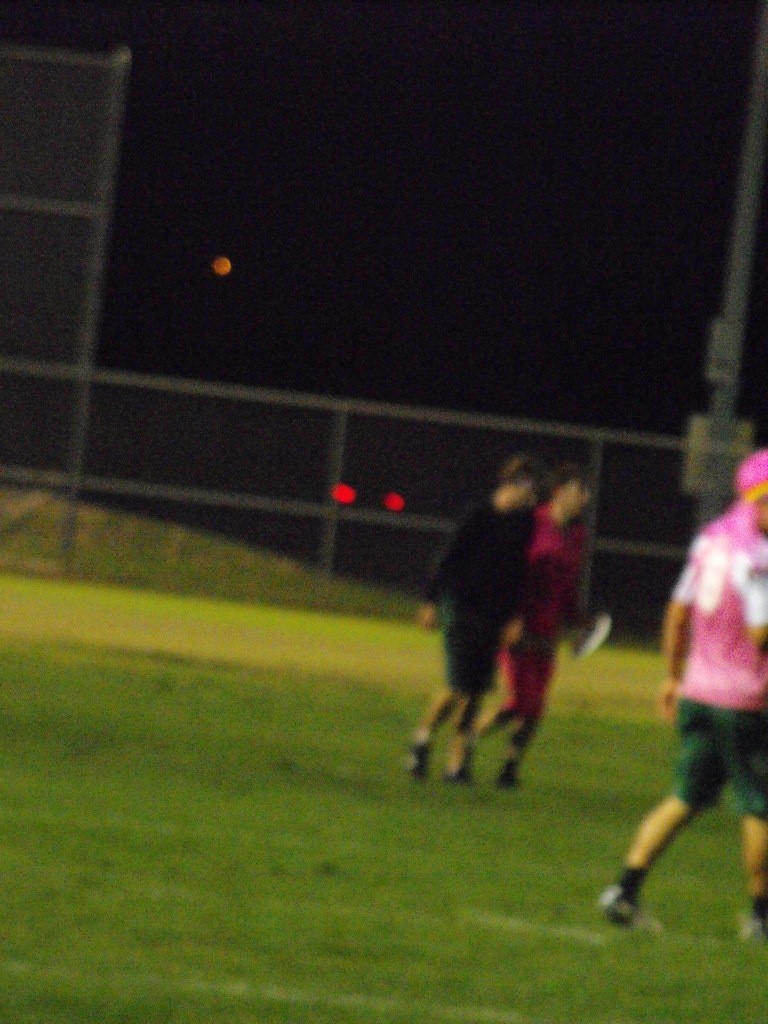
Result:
[478,464,596,788]
[600,448,768,935]
[408,451,539,785]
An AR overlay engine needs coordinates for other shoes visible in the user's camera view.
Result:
[601,886,637,927]
[735,918,767,940]
[445,766,467,788]
[494,761,516,790]
[405,741,429,781]
